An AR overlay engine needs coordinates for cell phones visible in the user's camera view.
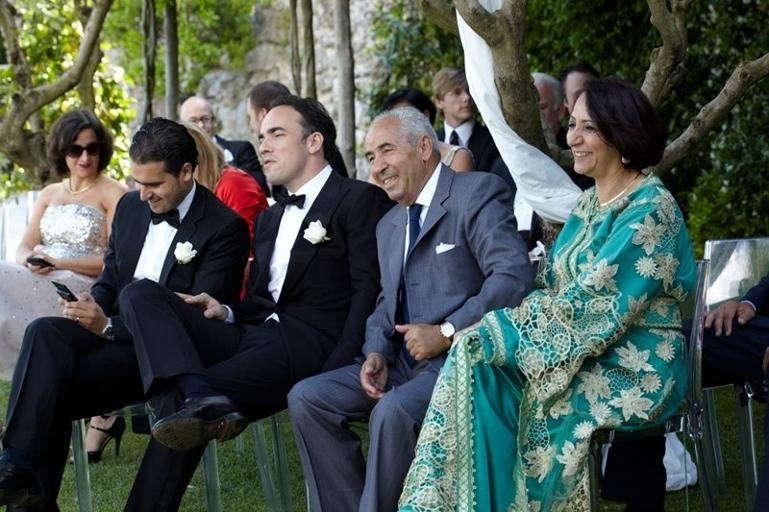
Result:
[52,281,78,302]
[26,257,55,268]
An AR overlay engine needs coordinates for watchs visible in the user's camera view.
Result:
[101,317,116,344]
[438,320,455,345]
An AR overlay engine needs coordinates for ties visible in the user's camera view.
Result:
[449,131,458,146]
[401,202,423,369]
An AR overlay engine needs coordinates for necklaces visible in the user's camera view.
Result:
[65,174,102,196]
[596,170,644,209]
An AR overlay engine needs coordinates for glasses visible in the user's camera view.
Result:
[66,141,99,158]
[188,114,214,124]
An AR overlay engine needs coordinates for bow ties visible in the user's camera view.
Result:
[150,208,180,229]
[272,191,306,209]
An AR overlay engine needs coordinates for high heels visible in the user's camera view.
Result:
[70,415,126,463]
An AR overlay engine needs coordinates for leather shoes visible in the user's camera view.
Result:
[0,465,45,509]
[151,394,248,452]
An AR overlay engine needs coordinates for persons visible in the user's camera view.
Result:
[287,105,534,512]
[529,73,571,150]
[245,79,292,139]
[0,109,132,469]
[102,94,397,512]
[175,119,269,303]
[397,82,699,512]
[599,271,769,512]
[0,117,252,512]
[430,65,500,172]
[562,62,601,115]
[380,86,475,174]
[178,95,271,204]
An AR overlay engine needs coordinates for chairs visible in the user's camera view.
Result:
[589,259,716,512]
[699,238,769,512]
